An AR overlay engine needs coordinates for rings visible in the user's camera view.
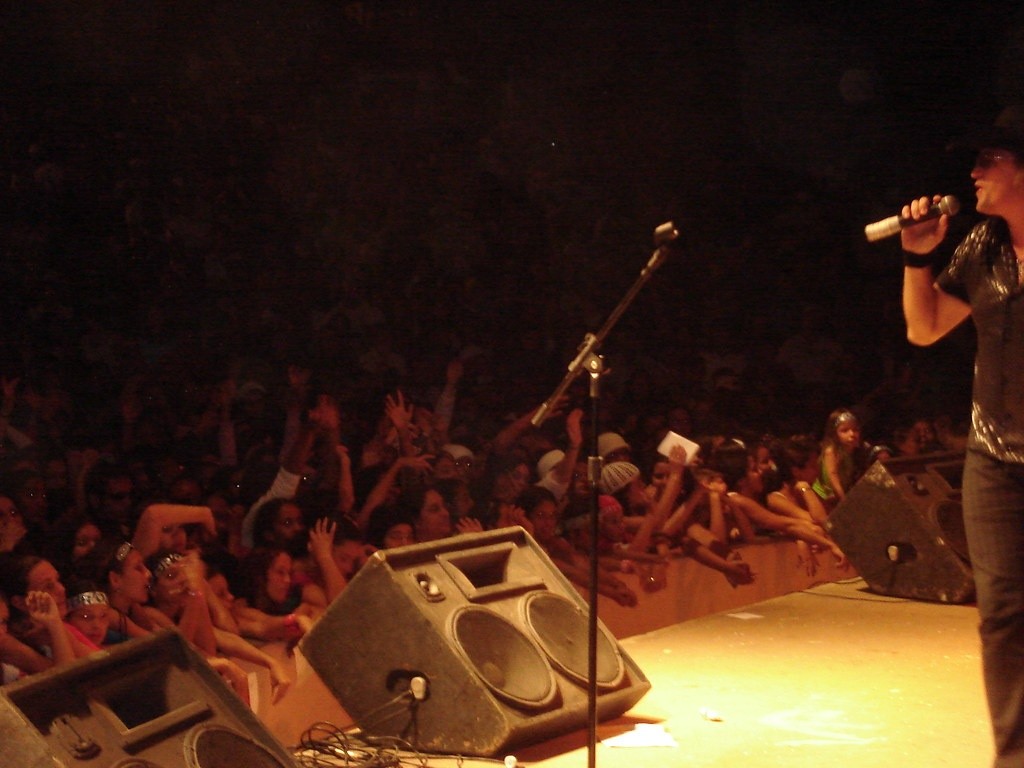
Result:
[651,577,654,582]
[677,456,679,458]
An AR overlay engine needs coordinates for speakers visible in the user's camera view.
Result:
[1,623,302,768]
[823,447,977,605]
[298,525,651,757]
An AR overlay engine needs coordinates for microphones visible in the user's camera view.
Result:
[857,195,962,245]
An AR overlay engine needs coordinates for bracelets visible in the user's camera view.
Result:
[902,249,931,268]
[187,591,203,597]
[800,487,811,495]
[285,614,295,626]
[620,559,629,572]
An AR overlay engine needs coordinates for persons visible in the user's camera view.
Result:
[901,104,1024,768]
[0,360,970,705]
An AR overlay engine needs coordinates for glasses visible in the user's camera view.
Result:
[103,488,136,502]
[975,152,1023,170]
[454,460,473,469]
[607,452,631,459]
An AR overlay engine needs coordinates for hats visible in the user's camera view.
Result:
[597,432,632,460]
[968,102,1024,162]
[441,445,474,461]
[532,447,564,480]
[601,462,640,496]
